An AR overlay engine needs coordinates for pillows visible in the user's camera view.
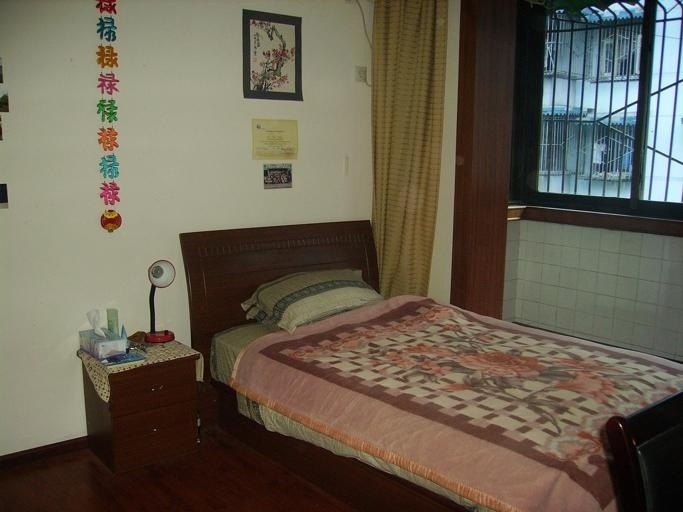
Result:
[240,269,384,332]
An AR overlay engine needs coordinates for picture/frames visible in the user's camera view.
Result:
[242,9,303,101]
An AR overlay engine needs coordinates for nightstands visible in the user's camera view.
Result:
[77,341,200,473]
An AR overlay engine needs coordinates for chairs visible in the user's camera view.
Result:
[606,393,683,511]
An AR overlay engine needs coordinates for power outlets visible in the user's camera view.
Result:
[355,66,367,83]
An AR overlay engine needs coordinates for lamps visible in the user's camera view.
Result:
[145,260,176,343]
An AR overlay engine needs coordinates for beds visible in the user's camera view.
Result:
[179,219,683,512]
